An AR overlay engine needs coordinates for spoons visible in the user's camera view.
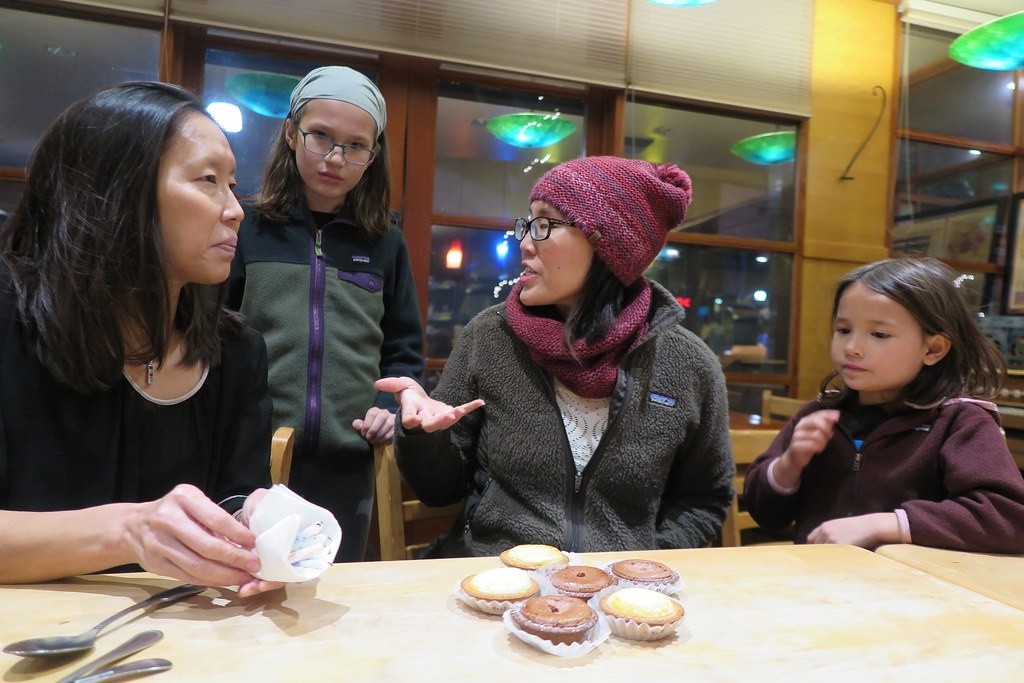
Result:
[3,583,207,658]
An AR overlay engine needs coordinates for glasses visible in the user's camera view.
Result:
[295,122,379,166]
[514,216,576,241]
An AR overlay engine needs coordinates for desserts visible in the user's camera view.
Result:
[510,594,598,646]
[612,559,673,584]
[599,588,684,626]
[500,544,569,570]
[550,565,612,601]
[461,567,539,603]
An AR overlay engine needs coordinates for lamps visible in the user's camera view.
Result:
[225,72,299,118]
[947,10,1024,71]
[729,126,797,166]
[485,109,578,149]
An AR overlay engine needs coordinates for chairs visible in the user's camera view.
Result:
[722,428,782,547]
[374,438,464,562]
[763,391,812,421]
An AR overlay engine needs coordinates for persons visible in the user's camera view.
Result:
[373,156,732,560]
[0,80,288,601]
[227,64,428,564]
[743,257,1024,557]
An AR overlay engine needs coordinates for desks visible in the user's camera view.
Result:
[0,541,1024,683]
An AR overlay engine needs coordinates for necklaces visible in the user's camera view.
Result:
[121,319,177,383]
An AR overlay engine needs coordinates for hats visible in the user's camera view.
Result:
[529,155,693,288]
